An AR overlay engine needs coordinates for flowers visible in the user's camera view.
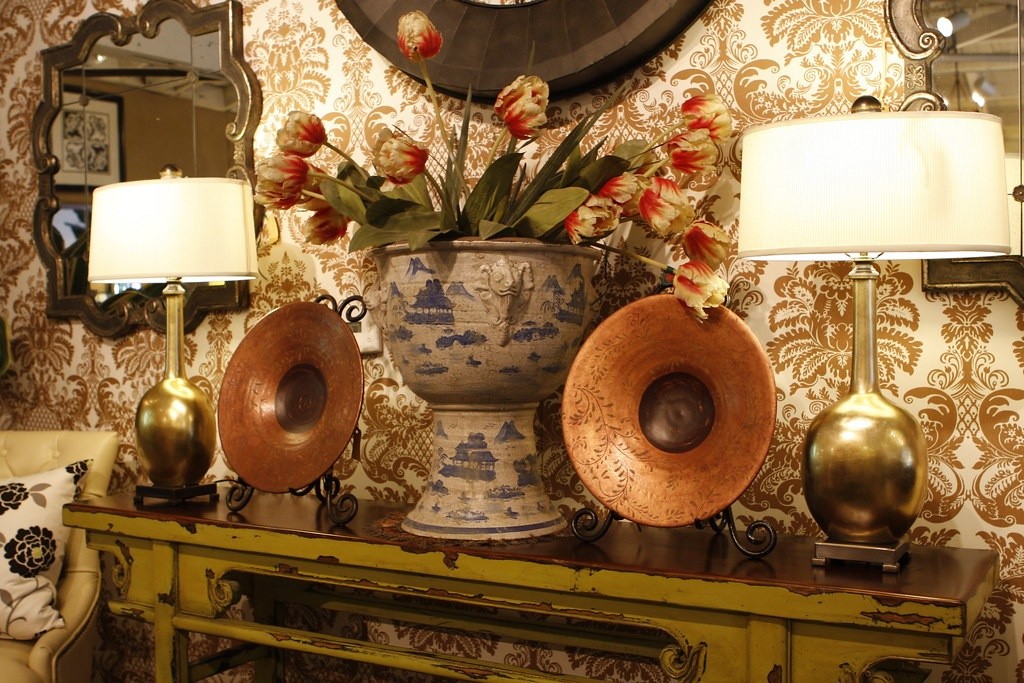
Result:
[253,12,733,325]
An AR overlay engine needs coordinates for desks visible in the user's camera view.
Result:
[62,485,1000,683]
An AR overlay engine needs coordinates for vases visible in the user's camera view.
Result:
[364,241,604,549]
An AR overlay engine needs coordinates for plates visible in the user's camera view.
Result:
[562,294,777,527]
[218,303,365,493]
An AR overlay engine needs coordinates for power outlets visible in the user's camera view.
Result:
[340,305,382,353]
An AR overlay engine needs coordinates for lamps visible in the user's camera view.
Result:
[87,163,259,513]
[936,10,972,38]
[974,78,997,98]
[738,112,1010,573]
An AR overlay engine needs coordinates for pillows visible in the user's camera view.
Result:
[0,458,94,641]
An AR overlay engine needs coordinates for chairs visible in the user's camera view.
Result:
[0,429,119,683]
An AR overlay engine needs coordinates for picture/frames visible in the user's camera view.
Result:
[50,84,125,194]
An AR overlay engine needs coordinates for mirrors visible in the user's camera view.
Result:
[29,0,268,341]
[883,0,1024,311]
